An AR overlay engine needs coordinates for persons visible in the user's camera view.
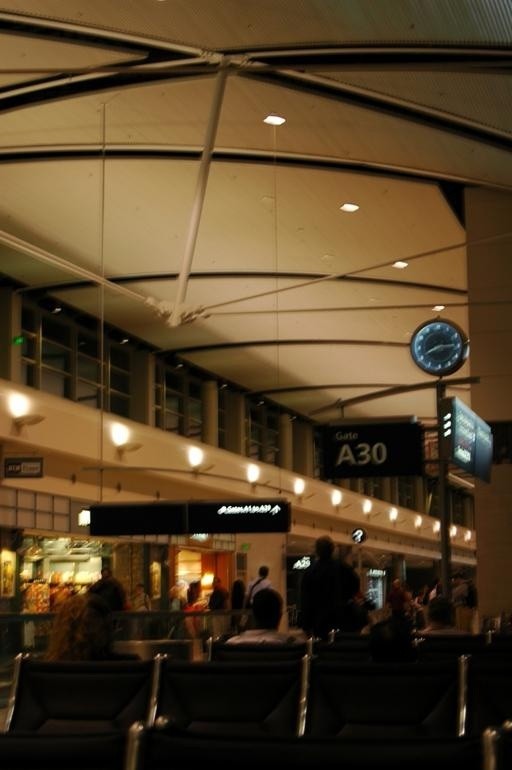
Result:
[43,536,479,660]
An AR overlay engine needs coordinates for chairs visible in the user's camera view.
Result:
[0,610,512,770]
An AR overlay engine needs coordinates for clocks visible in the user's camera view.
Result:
[410,314,468,376]
